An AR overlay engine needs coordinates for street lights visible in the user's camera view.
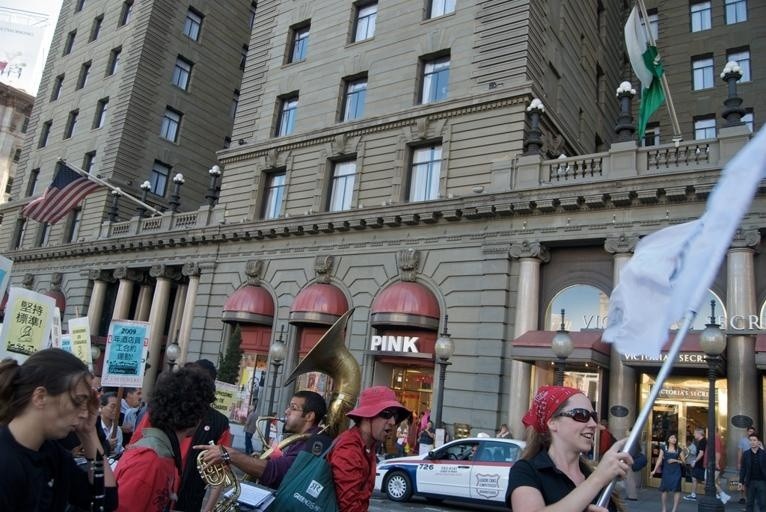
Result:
[273,449,340,512]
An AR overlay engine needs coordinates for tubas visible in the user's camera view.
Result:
[197,440,241,512]
[241,308,361,481]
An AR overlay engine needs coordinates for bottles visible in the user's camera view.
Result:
[345,384,414,424]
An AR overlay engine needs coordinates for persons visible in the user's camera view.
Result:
[129,358,232,512]
[497,423,514,439]
[192,392,327,491]
[505,384,633,512]
[417,421,436,456]
[112,369,215,512]
[325,386,411,511]
[397,419,409,458]
[648,427,765,512]
[471,443,484,461]
[58,383,148,497]
[0,346,119,512]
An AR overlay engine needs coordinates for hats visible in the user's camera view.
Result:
[374,432,526,509]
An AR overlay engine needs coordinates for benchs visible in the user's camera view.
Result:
[20,159,103,226]
[623,5,665,144]
[601,124,765,361]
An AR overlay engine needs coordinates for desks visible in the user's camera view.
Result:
[262,323,286,450]
[91,343,99,376]
[700,300,726,511]
[432,314,453,431]
[550,308,572,385]
[165,328,179,375]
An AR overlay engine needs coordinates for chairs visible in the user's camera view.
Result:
[683,494,698,501]
[720,495,731,504]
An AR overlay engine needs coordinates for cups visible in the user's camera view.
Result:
[554,407,600,423]
[288,403,315,413]
[471,450,477,452]
[377,409,401,419]
